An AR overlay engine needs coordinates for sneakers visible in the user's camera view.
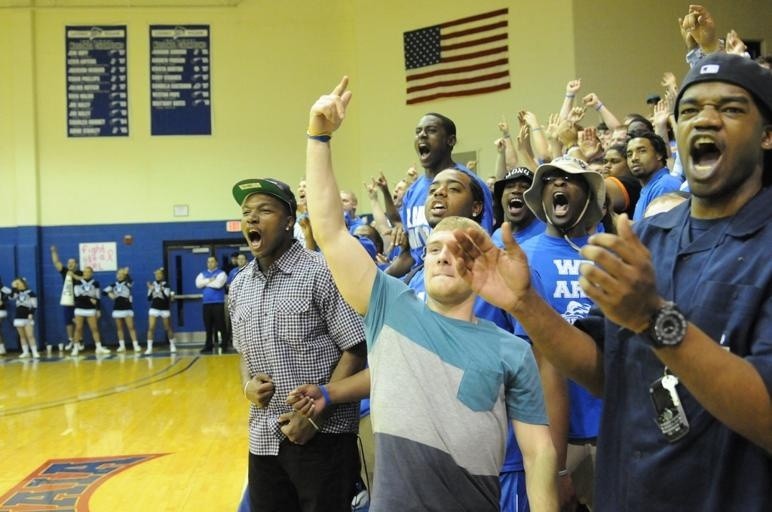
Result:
[201,344,213,353]
[170,344,176,352]
[19,353,31,359]
[78,339,85,351]
[33,352,41,359]
[72,350,79,357]
[145,349,153,355]
[134,346,142,352]
[117,347,126,353]
[65,339,73,350]
[96,347,112,354]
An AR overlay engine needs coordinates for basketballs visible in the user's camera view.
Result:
[123,234,132,245]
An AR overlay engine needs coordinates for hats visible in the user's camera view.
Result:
[675,53,772,120]
[494,167,538,222]
[523,157,607,228]
[233,178,297,216]
[647,96,660,104]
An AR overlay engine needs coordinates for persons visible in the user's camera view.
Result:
[0,281,12,356]
[8,278,40,358]
[219,252,240,346]
[144,267,177,354]
[102,267,141,352]
[70,266,111,356]
[50,246,85,351]
[229,5,771,512]
[195,256,227,353]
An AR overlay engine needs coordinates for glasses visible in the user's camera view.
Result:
[542,172,580,182]
